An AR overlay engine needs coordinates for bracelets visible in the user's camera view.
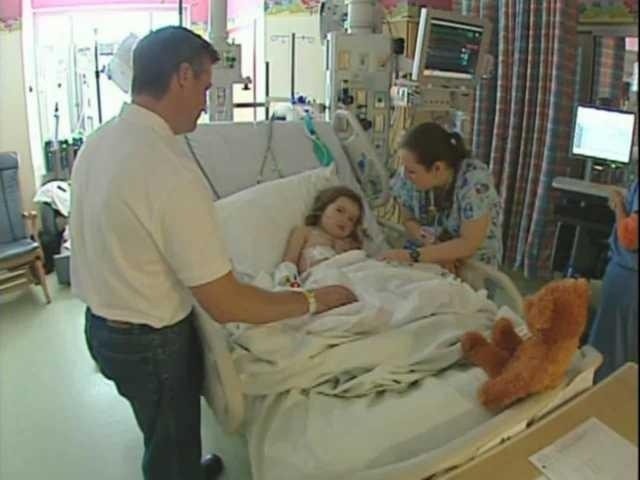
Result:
[302,286,318,315]
[410,243,421,264]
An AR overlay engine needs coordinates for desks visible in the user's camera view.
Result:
[441,362,640,479]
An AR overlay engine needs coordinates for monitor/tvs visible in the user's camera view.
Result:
[422,20,485,79]
[568,102,637,167]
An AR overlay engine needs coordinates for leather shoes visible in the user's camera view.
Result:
[195,454,222,479]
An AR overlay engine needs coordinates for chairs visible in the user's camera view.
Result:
[0,150,53,306]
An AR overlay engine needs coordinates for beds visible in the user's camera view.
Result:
[174,105,607,478]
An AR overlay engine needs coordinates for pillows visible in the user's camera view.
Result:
[212,157,338,279]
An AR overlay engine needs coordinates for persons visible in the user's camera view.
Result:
[588,166,640,387]
[69,26,359,480]
[376,122,504,300]
[280,185,372,274]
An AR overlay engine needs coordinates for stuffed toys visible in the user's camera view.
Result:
[462,275,591,416]
